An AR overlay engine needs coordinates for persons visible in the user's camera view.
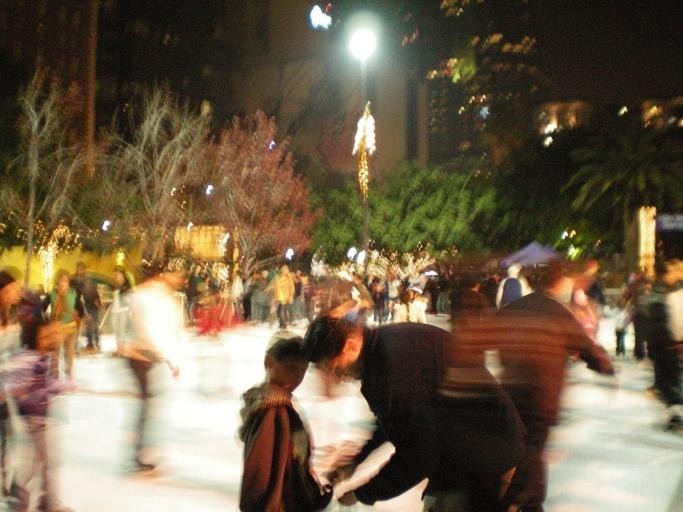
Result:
[0,255,683,511]
